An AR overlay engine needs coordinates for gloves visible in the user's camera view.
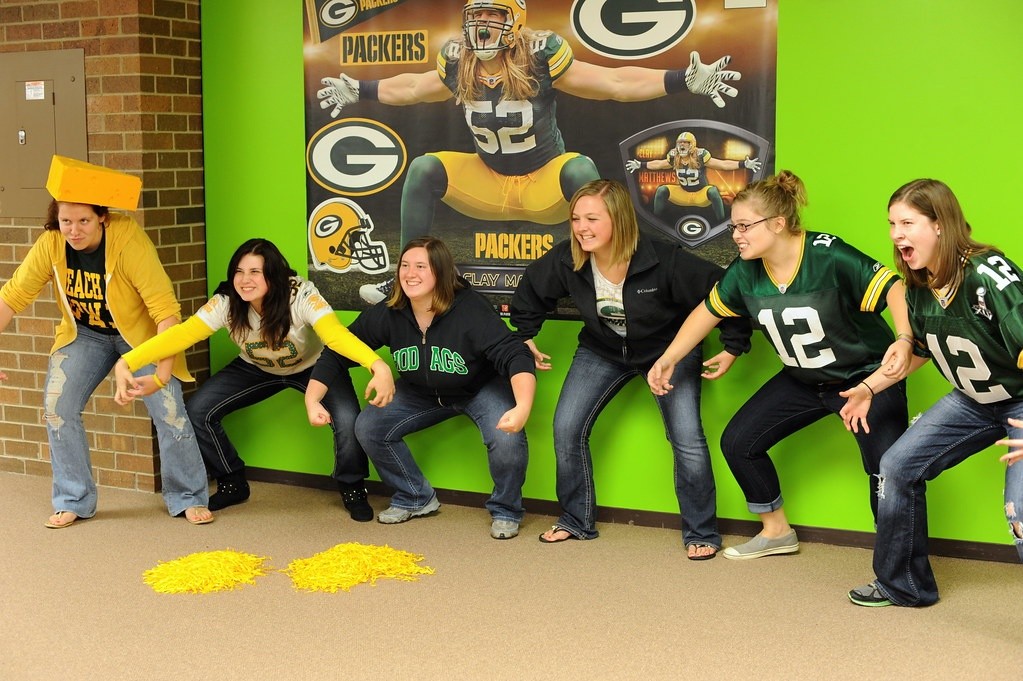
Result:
[745,156,762,173]
[625,158,641,173]
[665,51,741,108]
[317,73,379,118]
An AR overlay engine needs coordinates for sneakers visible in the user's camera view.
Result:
[377,496,441,524]
[491,519,518,539]
[359,277,396,305]
[723,528,799,560]
[848,582,894,607]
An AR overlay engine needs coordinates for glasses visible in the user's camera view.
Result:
[727,216,777,235]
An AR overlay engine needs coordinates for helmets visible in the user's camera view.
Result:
[676,132,696,156]
[463,0,527,48]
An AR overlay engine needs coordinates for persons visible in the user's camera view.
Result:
[112,238,396,522]
[304,235,538,540]
[316,0,742,257]
[510,178,753,561]
[0,199,214,526]
[838,178,1023,607]
[626,131,762,225]
[647,169,912,561]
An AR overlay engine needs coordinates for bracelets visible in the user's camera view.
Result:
[897,338,912,345]
[861,381,874,396]
[153,372,166,388]
[897,333,912,339]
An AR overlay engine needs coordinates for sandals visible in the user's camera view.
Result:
[45,512,73,528]
[688,544,716,560]
[539,526,575,543]
[185,507,214,524]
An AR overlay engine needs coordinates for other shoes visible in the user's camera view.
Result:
[339,479,373,522]
[208,481,250,511]
